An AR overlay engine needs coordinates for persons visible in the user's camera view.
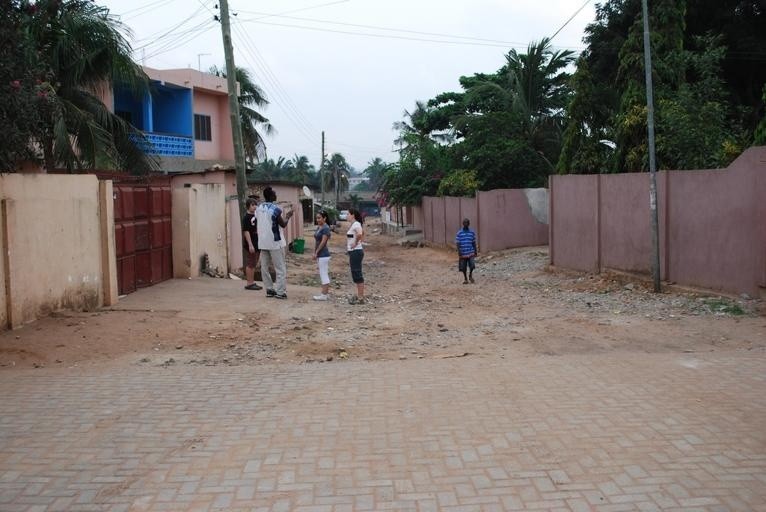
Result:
[241,198,263,291]
[312,208,334,302]
[255,186,295,300]
[345,208,366,305]
[454,218,478,284]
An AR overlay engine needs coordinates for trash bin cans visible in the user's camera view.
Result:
[293,239,304,253]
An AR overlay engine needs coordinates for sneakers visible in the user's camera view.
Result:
[266,289,287,298]
[464,276,474,284]
[347,295,364,304]
[313,294,329,301]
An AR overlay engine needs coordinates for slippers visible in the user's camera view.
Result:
[244,283,261,290]
